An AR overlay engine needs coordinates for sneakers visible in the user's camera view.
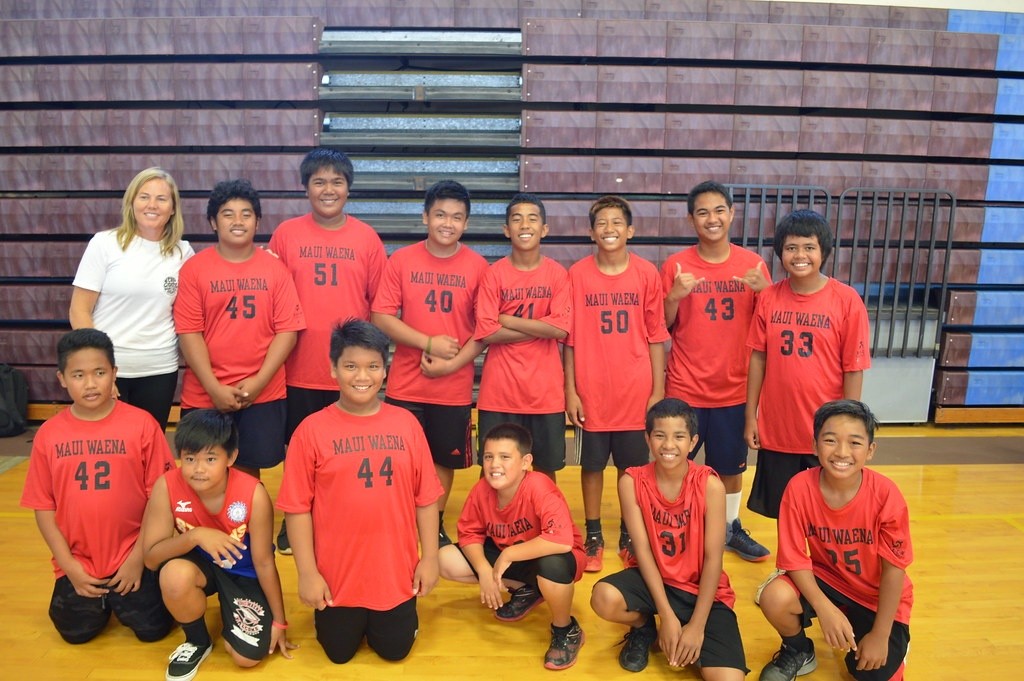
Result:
[760,631,817,680]
[613,612,658,672]
[544,616,585,670]
[438,527,451,547]
[723,519,771,562]
[617,535,638,569]
[167,636,213,680]
[495,582,544,621]
[276,515,293,556]
[582,531,604,572]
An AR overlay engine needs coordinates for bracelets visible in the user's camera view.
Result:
[272,620,288,629]
[426,336,432,354]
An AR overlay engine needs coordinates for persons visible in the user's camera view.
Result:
[275,320,445,663]
[371,181,490,547]
[69,168,279,434]
[564,197,671,572]
[142,408,295,681]
[173,182,307,478]
[438,424,586,670]
[659,181,773,562]
[590,398,751,681]
[268,149,388,555]
[21,329,175,644]
[743,210,871,604]
[474,195,572,484]
[758,399,913,681]
